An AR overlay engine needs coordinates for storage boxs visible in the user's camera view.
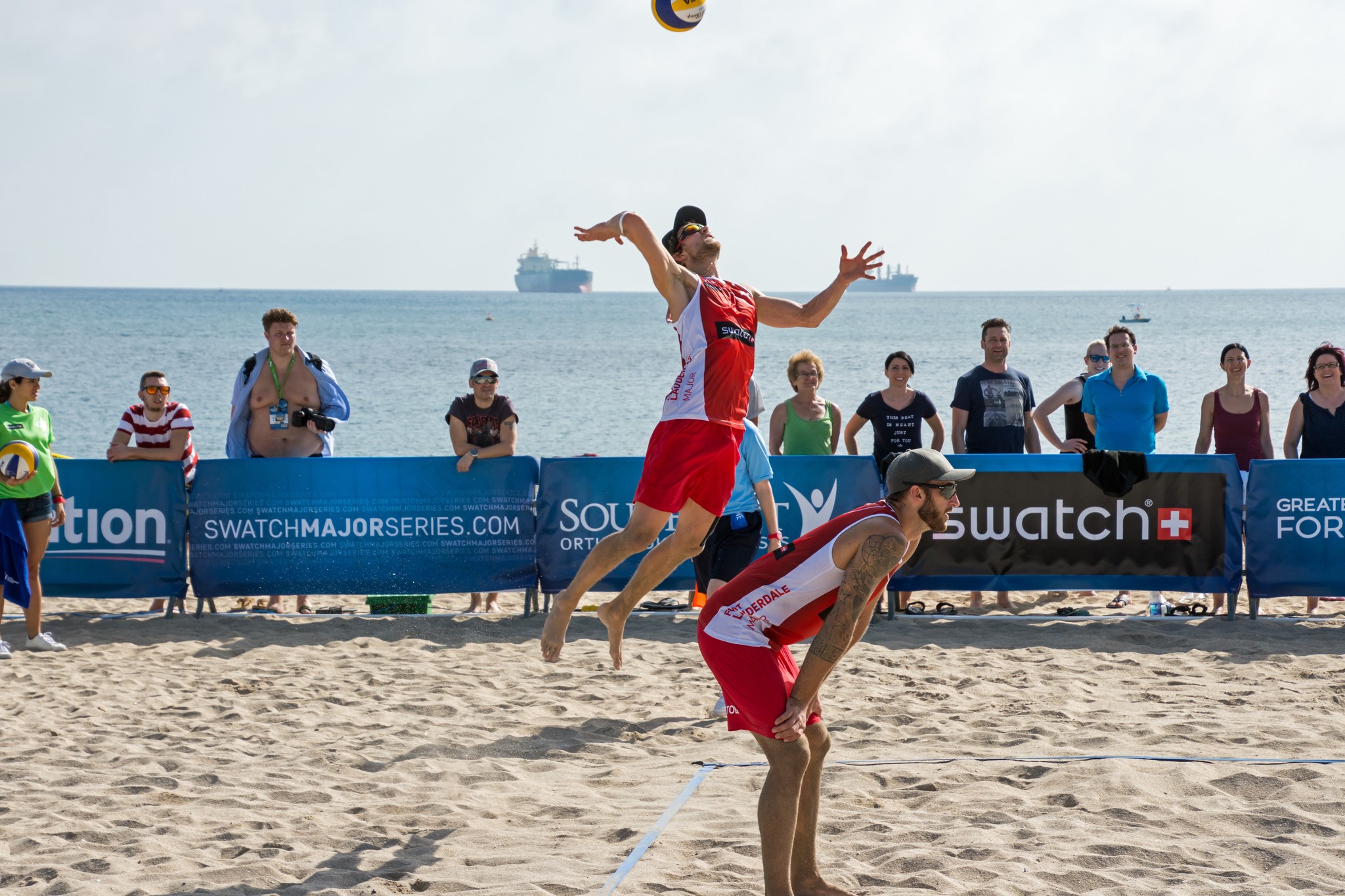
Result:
[365,594,432,614]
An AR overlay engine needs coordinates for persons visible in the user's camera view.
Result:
[950,318,1041,611]
[106,370,199,614]
[689,417,783,604]
[540,205,886,669]
[1031,339,1113,598]
[1194,343,1285,616]
[746,374,766,427]
[0,358,69,660]
[768,349,841,456]
[1283,341,1345,615]
[698,448,976,896]
[226,307,350,615]
[445,357,518,614]
[844,351,945,612]
[1082,324,1175,610]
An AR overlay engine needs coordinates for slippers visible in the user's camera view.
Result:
[632,597,689,612]
[296,606,316,614]
[252,598,269,609]
[906,601,926,615]
[231,597,255,611]
[1106,597,1129,608]
[1055,607,1090,616]
[264,606,286,614]
[1164,603,1210,616]
[1321,596,1345,601]
[1179,591,1209,604]
[932,602,958,615]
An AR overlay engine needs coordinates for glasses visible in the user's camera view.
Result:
[471,376,497,384]
[142,386,170,395]
[1108,342,1134,349]
[676,223,705,251]
[1086,355,1111,363]
[1314,362,1340,369]
[902,481,957,500]
[796,371,820,377]
[275,398,287,421]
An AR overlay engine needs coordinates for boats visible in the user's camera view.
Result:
[845,246,919,293]
[513,237,594,293]
[1118,302,1151,323]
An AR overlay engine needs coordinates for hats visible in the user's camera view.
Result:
[662,206,707,258]
[1,358,52,383]
[470,357,499,378]
[885,448,976,493]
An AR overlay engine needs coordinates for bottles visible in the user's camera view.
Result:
[1147,605,1179,616]
[1148,591,1162,617]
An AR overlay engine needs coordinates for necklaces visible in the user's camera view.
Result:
[797,394,817,409]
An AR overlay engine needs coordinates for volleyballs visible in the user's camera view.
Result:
[650,0,706,32]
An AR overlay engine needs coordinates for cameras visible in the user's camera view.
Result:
[290,406,337,432]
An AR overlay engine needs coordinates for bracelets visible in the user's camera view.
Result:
[618,211,633,237]
[52,497,65,503]
[6,478,13,487]
[53,494,63,499]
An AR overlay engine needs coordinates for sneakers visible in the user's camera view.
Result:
[0,639,13,659]
[25,631,68,651]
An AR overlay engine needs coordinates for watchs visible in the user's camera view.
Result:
[768,531,782,540]
[470,448,479,460]
